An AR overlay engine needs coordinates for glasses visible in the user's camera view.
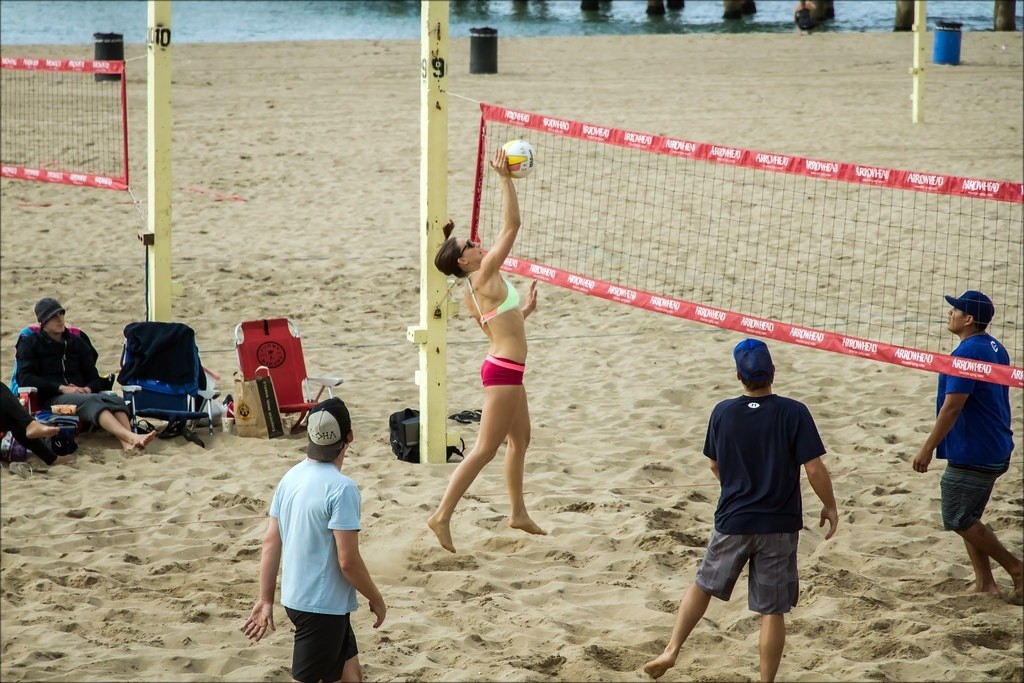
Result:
[459,239,475,258]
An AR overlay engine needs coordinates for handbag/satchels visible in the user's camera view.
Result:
[232,366,285,438]
[0,431,27,462]
[35,412,77,455]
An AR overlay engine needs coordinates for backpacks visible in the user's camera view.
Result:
[389,407,465,463]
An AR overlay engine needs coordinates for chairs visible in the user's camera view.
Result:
[9,325,115,433]
[119,321,221,434]
[234,317,343,429]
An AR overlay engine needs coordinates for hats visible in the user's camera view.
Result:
[307,398,352,460]
[35,297,66,327]
[733,338,773,380]
[944,291,995,323]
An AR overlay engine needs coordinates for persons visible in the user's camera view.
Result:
[427,146,548,554]
[17,297,157,451]
[0,381,76,467]
[913,289,1024,593]
[242,396,386,683]
[642,337,839,683]
[793,0,815,35]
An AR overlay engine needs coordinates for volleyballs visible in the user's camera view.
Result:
[502,140,536,179]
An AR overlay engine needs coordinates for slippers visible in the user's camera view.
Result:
[10,467,49,473]
[10,462,32,478]
[461,409,482,414]
[449,414,479,424]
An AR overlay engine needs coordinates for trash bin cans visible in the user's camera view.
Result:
[93,32,124,82]
[932,21,964,65]
[469,27,498,74]
